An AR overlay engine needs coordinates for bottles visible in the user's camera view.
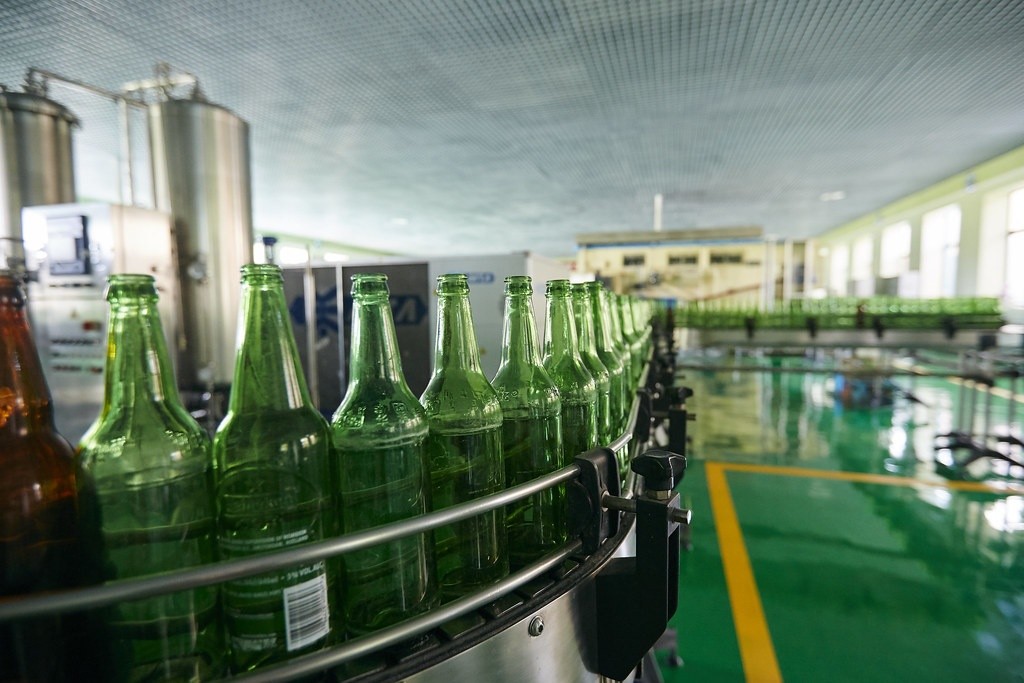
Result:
[490,276,569,576]
[77,274,226,682]
[418,273,512,609]
[331,273,434,666]
[571,280,1002,494]
[541,280,597,536]
[1,269,116,683]
[210,264,344,682]
